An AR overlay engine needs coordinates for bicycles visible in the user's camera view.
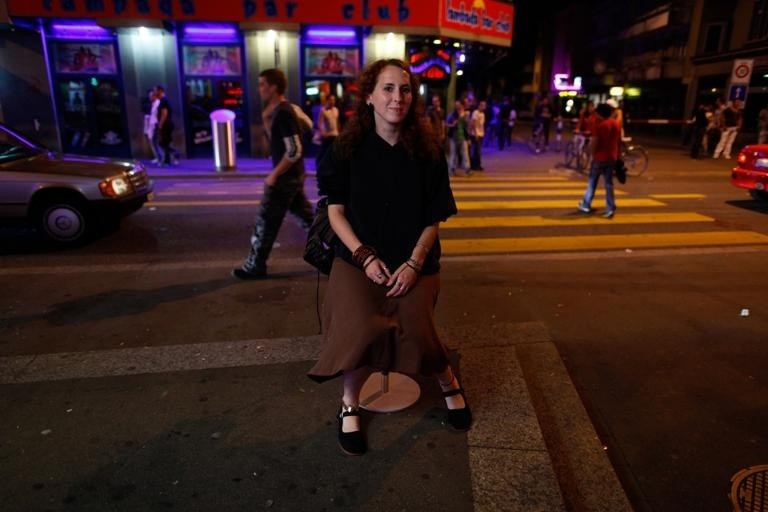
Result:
[618,136,649,179]
[524,129,546,154]
[563,132,592,170]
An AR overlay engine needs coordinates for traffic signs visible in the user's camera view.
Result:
[728,58,756,85]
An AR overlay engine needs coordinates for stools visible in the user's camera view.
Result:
[352,290,422,414]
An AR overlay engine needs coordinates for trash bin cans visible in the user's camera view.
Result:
[209,109,237,172]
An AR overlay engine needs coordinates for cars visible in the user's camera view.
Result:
[730,142,768,206]
[0,125,156,247]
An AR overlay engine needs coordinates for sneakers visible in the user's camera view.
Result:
[577,202,591,213]
[712,153,720,159]
[602,210,614,218]
[722,152,731,160]
[231,268,250,280]
[336,403,368,457]
[442,378,473,430]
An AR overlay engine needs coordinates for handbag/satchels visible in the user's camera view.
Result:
[302,132,355,276]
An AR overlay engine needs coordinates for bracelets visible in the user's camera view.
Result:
[405,256,421,273]
[353,243,377,270]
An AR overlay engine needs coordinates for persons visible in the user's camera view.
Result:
[425,94,487,176]
[231,68,320,280]
[572,98,626,217]
[318,93,342,143]
[153,85,181,169]
[494,95,518,150]
[758,104,767,147]
[320,58,473,458]
[146,92,175,168]
[691,98,742,158]
[534,94,552,146]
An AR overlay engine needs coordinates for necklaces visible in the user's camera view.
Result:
[376,125,400,144]
